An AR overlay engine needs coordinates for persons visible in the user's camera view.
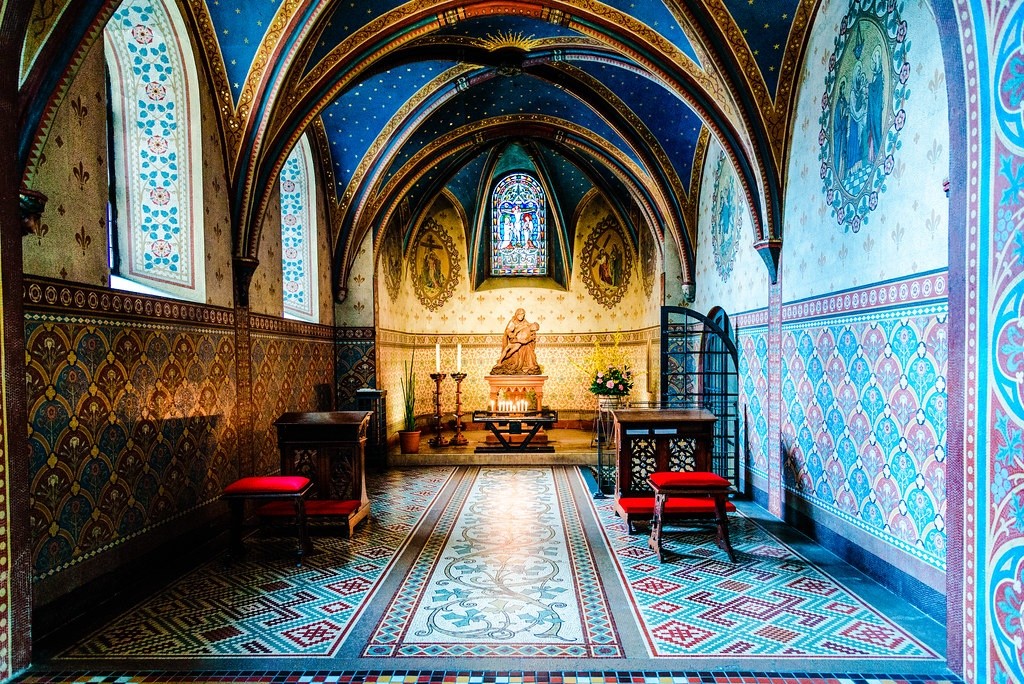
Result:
[493,322,540,369]
[494,308,538,369]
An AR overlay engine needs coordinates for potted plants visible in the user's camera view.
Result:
[398,341,421,454]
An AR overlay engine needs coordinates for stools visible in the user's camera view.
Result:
[646,472,735,563]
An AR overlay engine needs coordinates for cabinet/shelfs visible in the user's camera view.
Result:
[255,411,374,537]
[615,409,737,533]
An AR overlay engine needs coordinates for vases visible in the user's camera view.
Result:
[598,394,619,412]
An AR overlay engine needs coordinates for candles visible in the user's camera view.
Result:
[435,343,441,373]
[457,343,461,372]
[489,399,529,412]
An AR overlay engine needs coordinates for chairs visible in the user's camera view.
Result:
[219,474,316,557]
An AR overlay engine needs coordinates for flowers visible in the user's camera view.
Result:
[589,364,633,396]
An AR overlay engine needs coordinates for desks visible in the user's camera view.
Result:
[473,409,559,454]
